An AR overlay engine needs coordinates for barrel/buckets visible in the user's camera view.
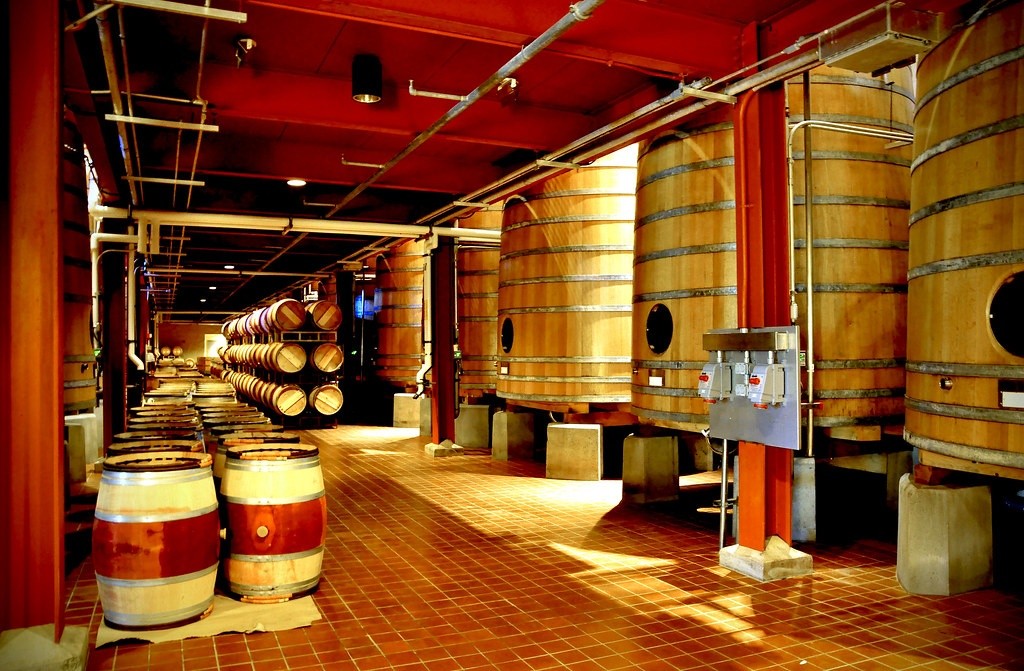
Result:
[91,367,327,632]
[210,299,344,417]
[161,346,183,356]
[185,358,195,368]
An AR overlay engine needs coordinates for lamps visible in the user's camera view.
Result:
[352,55,383,103]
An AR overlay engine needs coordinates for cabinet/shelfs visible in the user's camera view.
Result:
[227,331,339,429]
